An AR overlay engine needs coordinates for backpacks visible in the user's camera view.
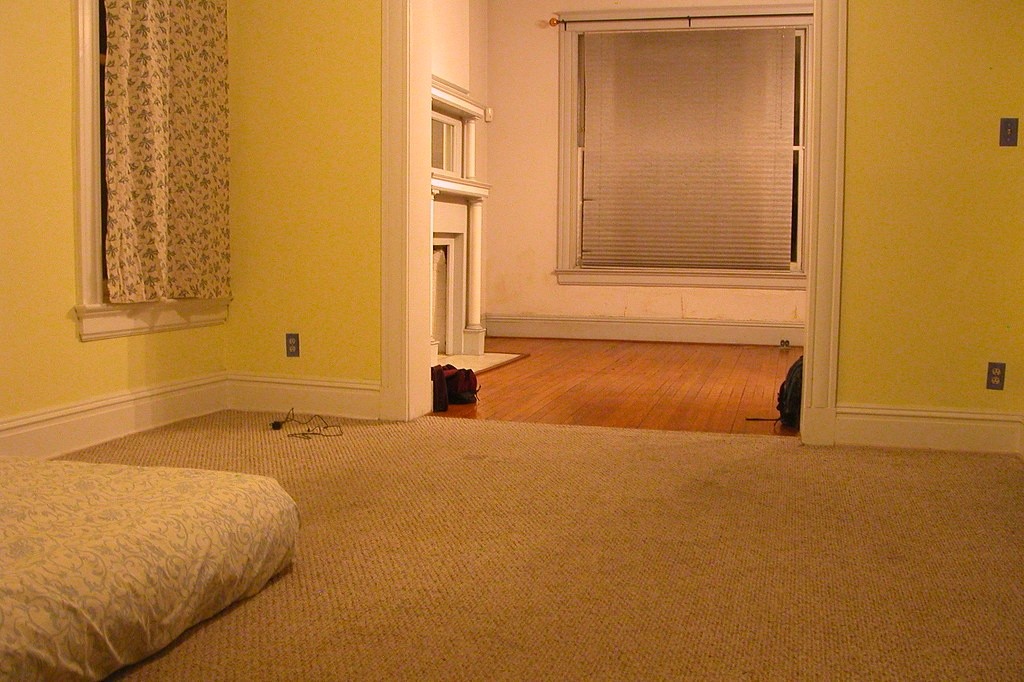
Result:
[776,355,803,431]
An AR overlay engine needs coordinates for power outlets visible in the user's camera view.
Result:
[286,334,299,357]
[986,363,1006,390]
